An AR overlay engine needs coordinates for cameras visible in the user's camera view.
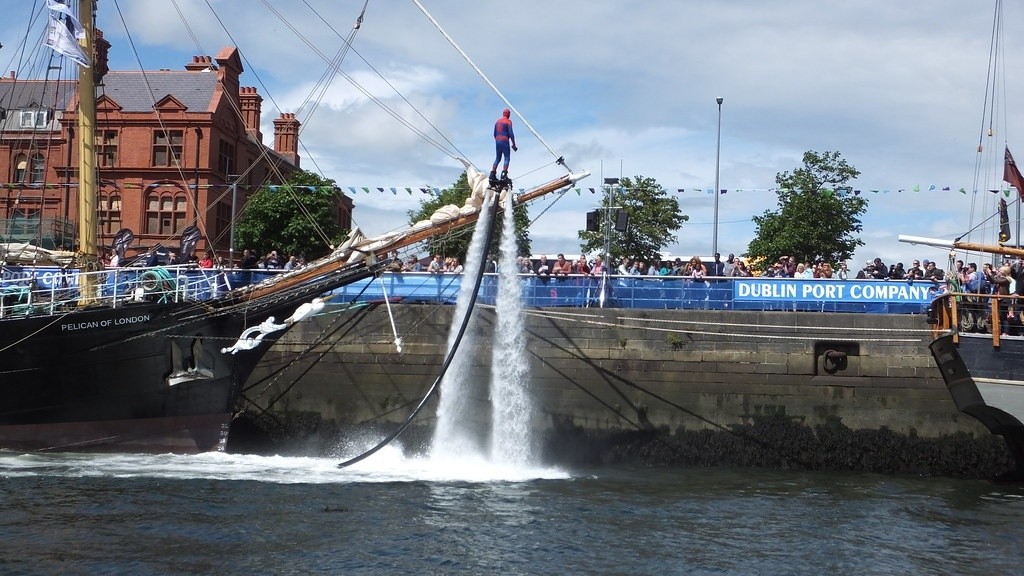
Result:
[784,258,789,263]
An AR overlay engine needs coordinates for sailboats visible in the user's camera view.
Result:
[896,1,1024,480]
[1,1,591,457]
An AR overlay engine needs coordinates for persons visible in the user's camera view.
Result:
[518,253,604,307]
[144,246,158,267]
[617,256,708,309]
[856,258,923,280]
[489,109,515,180]
[374,250,465,306]
[184,250,227,299]
[713,253,748,277]
[924,260,1024,304]
[101,249,120,267]
[241,250,305,287]
[761,256,848,279]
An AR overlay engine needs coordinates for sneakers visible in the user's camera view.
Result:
[501,171,509,179]
[489,171,499,180]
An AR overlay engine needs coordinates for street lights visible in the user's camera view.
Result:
[711,96,724,258]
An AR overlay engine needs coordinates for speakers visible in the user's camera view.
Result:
[615,212,629,232]
[587,211,600,232]
